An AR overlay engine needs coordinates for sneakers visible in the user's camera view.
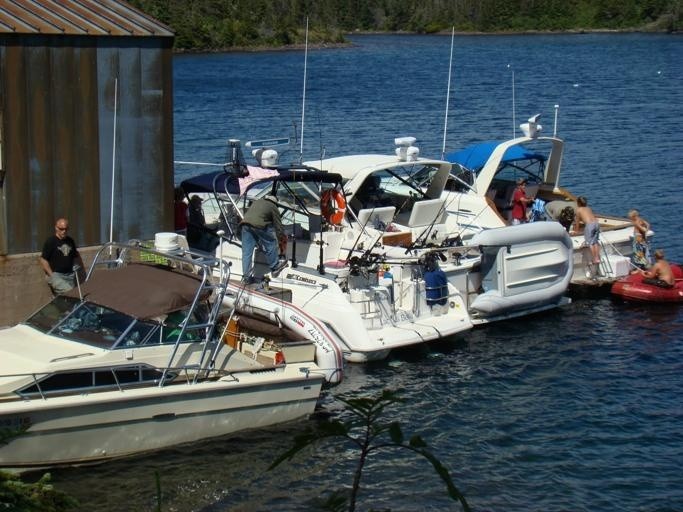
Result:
[242,276,254,285]
[273,259,288,274]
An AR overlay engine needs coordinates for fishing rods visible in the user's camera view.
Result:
[293,114,497,277]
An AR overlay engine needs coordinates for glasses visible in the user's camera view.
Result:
[57,226,68,231]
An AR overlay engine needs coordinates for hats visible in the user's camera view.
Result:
[266,196,277,204]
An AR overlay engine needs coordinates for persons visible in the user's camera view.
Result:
[569,196,600,264]
[511,177,534,226]
[641,250,675,288]
[238,195,288,285]
[628,210,649,275]
[39,219,78,295]
[174,187,220,253]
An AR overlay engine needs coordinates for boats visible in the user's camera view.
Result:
[189,20,653,367]
[611,260,682,304]
[206,273,344,393]
[0,77,343,471]
